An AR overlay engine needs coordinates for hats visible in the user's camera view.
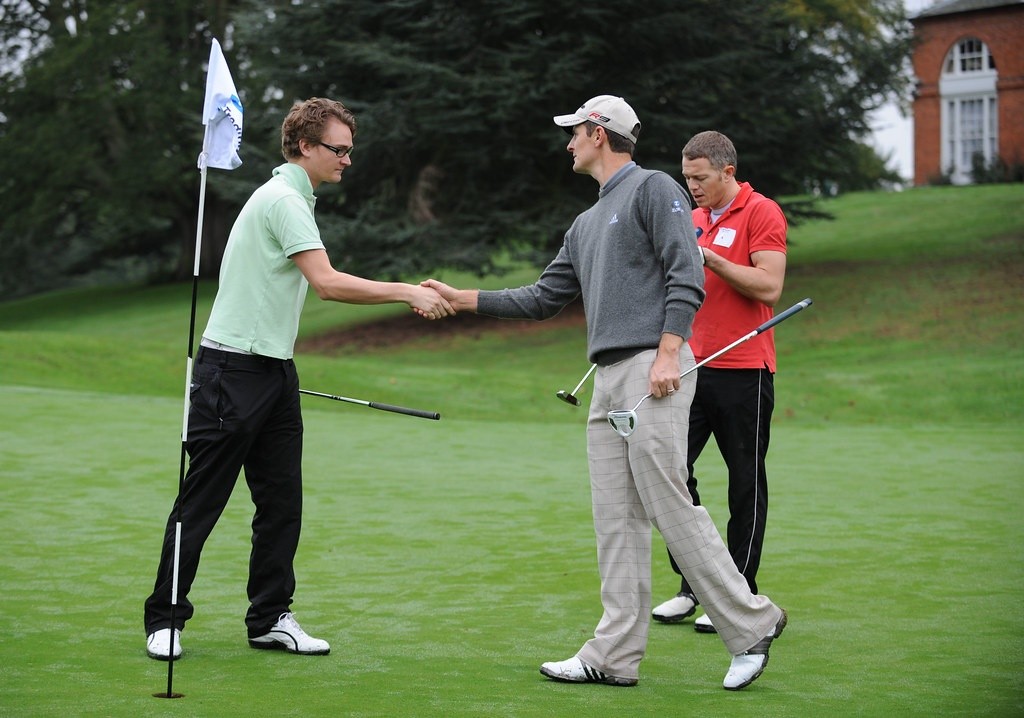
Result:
[553,95,642,144]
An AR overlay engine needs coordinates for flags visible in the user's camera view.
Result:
[197,37,243,171]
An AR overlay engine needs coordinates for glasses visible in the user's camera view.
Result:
[318,142,355,158]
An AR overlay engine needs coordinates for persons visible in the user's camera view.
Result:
[144,97,457,660]
[412,95,787,691]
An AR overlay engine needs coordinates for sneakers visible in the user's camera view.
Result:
[248,611,332,655]
[723,609,788,691]
[650,592,700,622]
[540,656,639,686]
[694,614,719,634]
[146,628,183,661]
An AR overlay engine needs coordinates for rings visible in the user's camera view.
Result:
[667,388,676,393]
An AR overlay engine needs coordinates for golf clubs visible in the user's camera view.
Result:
[299,384,443,422]
[557,363,598,407]
[604,296,811,435]
